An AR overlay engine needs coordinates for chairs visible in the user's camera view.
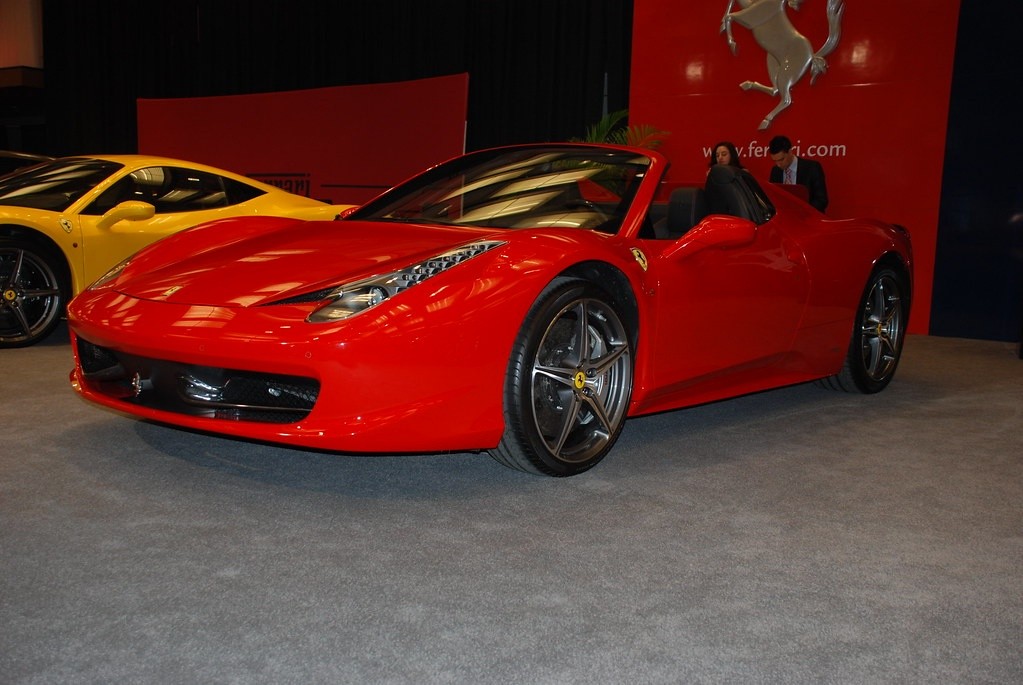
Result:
[647,166,763,242]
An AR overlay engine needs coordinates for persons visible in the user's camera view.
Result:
[708,141,744,171]
[768,135,829,214]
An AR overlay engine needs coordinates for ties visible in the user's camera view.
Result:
[784,168,792,186]
